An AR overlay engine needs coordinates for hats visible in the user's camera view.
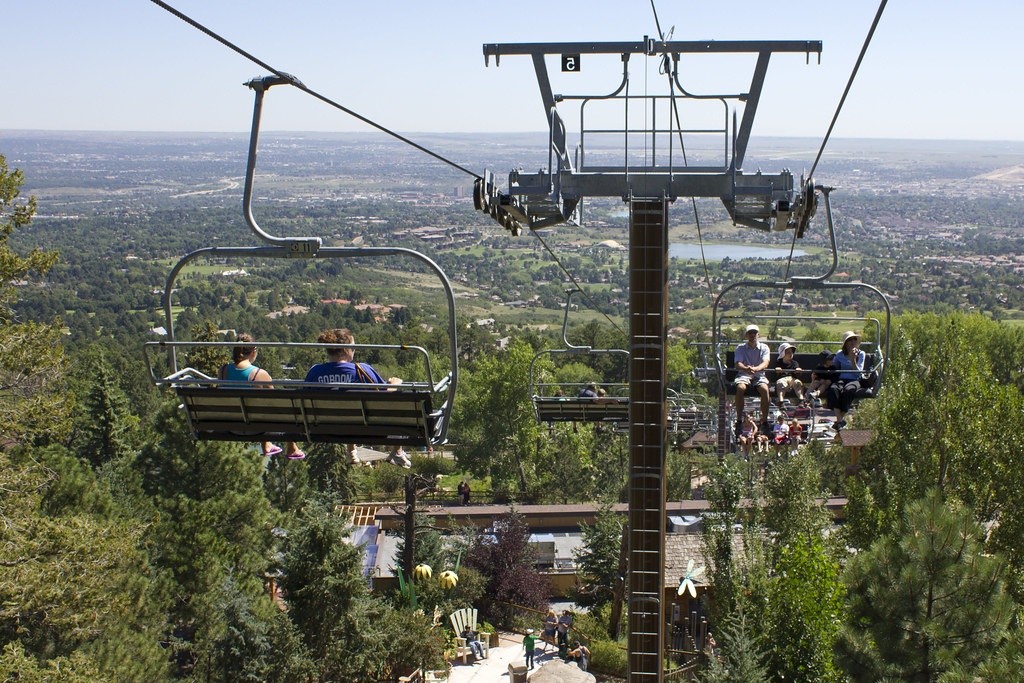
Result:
[526,629,533,634]
[777,343,796,362]
[746,325,759,332]
[818,350,836,366]
[842,331,860,347]
[561,643,565,645]
[465,625,470,629]
[574,641,580,646]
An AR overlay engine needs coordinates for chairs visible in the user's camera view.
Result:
[449,607,491,664]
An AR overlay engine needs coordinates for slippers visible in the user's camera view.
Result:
[285,451,305,459]
[260,445,282,455]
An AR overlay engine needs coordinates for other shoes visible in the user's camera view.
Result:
[791,451,794,456]
[764,428,774,438]
[832,421,846,429]
[809,392,818,399]
[735,423,742,434]
[794,450,798,455]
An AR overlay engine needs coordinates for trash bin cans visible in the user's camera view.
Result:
[507,662,528,683]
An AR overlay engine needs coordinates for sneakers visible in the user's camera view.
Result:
[386,452,411,469]
[347,450,360,463]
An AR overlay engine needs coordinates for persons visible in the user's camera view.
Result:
[734,325,771,433]
[810,331,865,429]
[705,633,716,655]
[676,400,803,463]
[523,628,544,669]
[460,625,487,660]
[304,327,412,470]
[548,382,608,436]
[772,343,807,410]
[799,348,838,401]
[215,333,306,459]
[458,481,471,506]
[544,610,590,670]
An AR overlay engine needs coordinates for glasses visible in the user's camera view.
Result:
[828,356,834,360]
[849,338,858,342]
